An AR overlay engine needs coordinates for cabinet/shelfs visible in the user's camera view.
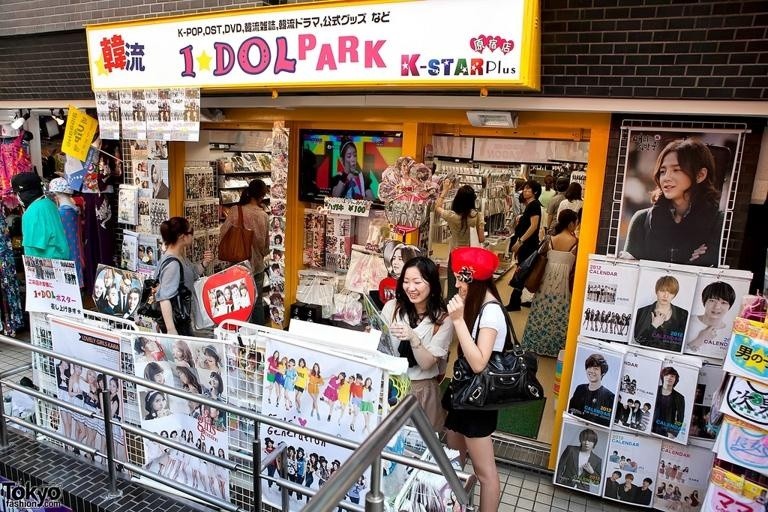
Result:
[218,171,273,205]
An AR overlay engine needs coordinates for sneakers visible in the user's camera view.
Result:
[504,306,521,311]
[521,302,531,307]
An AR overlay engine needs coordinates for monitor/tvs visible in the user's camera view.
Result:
[298,129,403,212]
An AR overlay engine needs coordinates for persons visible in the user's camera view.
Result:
[26,258,377,512]
[382,242,417,306]
[327,134,377,201]
[148,216,217,336]
[106,87,218,258]
[272,217,285,235]
[502,179,541,311]
[268,249,283,264]
[215,179,269,326]
[301,211,352,271]
[442,246,508,512]
[553,273,737,512]
[12,170,69,288]
[231,157,246,171]
[520,208,579,357]
[272,235,283,248]
[47,178,83,292]
[235,156,251,171]
[270,149,289,216]
[622,138,723,266]
[435,177,487,305]
[381,257,455,440]
[507,173,583,250]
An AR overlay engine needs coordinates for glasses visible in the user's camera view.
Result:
[184,228,193,236]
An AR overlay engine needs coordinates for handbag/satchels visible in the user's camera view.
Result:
[508,234,527,253]
[508,250,539,290]
[218,226,254,263]
[194,279,218,328]
[469,227,482,247]
[449,350,544,411]
[138,279,192,321]
[524,254,548,293]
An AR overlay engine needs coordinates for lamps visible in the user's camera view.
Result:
[465,110,518,131]
[8,109,64,130]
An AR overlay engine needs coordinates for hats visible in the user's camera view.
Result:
[450,247,500,281]
[11,171,41,192]
[45,177,74,195]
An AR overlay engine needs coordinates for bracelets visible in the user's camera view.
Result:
[516,237,523,246]
[411,338,422,349]
[439,195,445,199]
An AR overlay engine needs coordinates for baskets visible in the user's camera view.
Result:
[382,429,404,475]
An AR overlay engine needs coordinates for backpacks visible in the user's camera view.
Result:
[433,312,451,385]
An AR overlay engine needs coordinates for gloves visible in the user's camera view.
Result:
[511,237,523,252]
